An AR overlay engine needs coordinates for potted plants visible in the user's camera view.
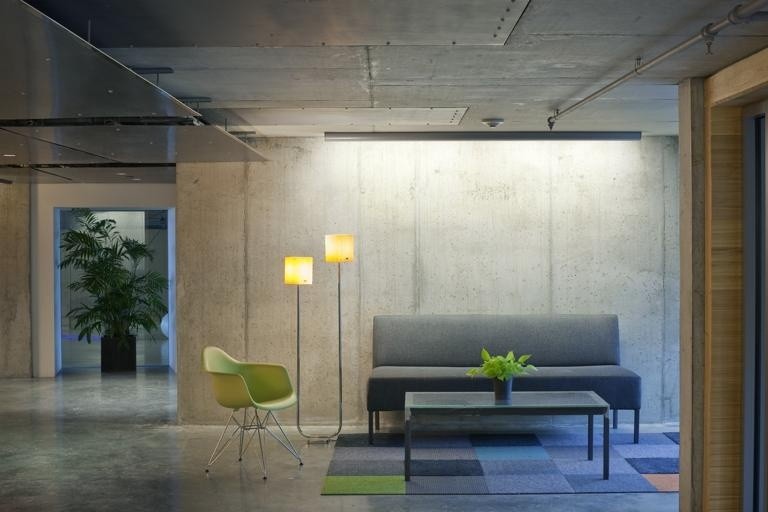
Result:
[57,207,168,373]
[468,347,536,400]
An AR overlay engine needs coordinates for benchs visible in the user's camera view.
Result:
[366,315,642,444]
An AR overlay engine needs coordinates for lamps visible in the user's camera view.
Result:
[283,233,355,446]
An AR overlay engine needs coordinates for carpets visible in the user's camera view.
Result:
[320,431,679,495]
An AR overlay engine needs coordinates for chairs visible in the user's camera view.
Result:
[202,345,302,481]
